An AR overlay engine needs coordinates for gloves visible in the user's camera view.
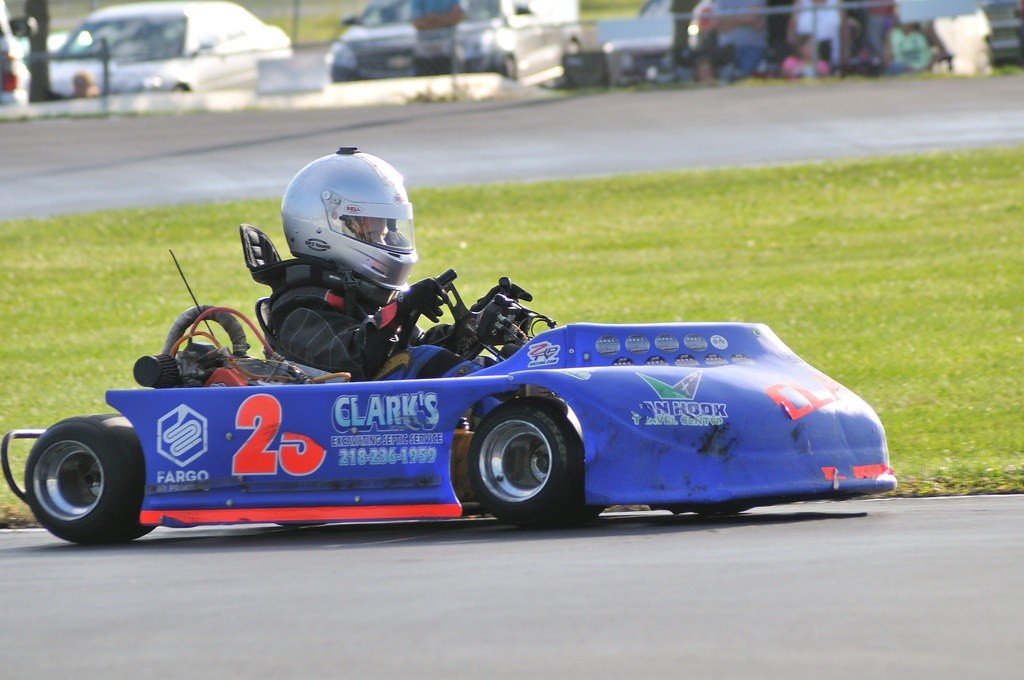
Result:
[388,277,449,323]
[470,283,533,312]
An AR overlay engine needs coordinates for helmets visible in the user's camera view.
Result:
[280,147,419,293]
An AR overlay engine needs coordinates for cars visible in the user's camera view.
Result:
[561,0,769,87]
[325,1,584,87]
[48,2,294,99]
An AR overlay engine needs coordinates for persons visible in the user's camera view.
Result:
[889,17,931,77]
[263,150,535,383]
[781,33,834,80]
